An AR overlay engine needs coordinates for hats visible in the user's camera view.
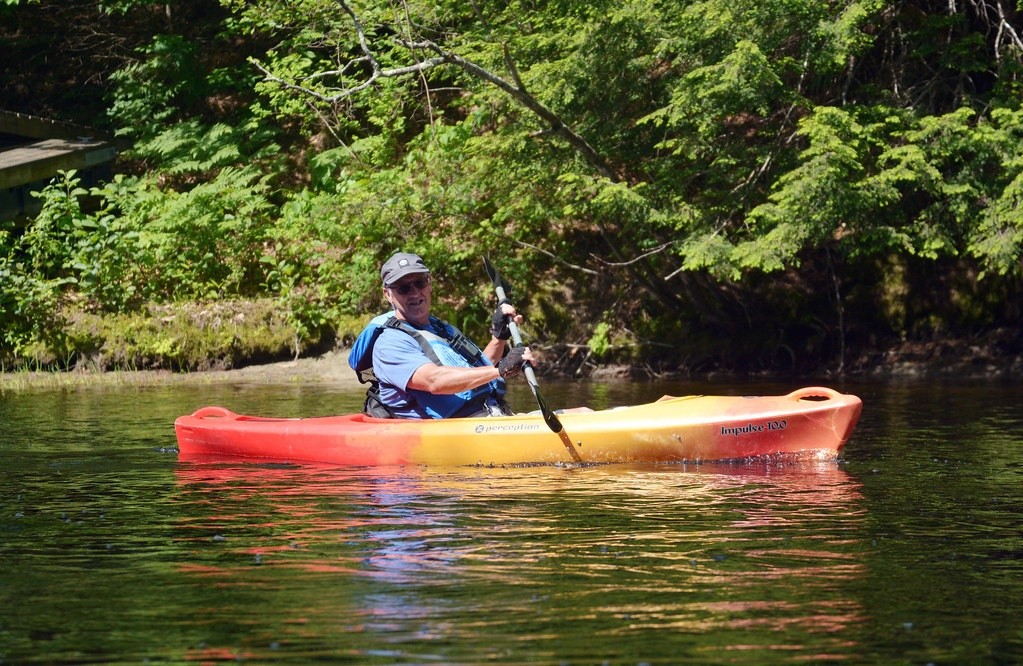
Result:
[380,252,430,285]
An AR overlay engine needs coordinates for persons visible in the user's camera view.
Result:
[372,252,537,419]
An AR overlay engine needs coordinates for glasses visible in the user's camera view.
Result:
[384,275,429,295]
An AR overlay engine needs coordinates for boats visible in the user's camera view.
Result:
[174,386,864,465]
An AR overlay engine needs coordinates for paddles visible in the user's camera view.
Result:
[482,254,563,435]
